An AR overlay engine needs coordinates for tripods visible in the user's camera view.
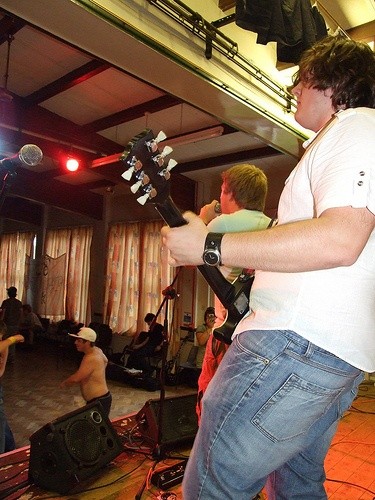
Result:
[124,266,190,500]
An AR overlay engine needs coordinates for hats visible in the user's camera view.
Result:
[68,327,97,343]
[6,287,18,292]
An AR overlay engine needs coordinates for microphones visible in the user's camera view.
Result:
[0,144,43,174]
[214,204,221,213]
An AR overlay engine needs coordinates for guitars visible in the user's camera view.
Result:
[118,128,255,345]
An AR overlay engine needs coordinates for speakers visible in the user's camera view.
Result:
[28,400,125,495]
[134,392,201,448]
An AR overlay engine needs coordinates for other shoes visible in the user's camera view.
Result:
[10,362,15,367]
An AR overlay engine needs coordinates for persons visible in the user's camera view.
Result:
[0,287,44,367]
[132,313,168,360]
[0,319,25,456]
[160,38,375,500]
[60,327,112,417]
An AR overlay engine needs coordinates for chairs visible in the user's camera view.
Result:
[33,317,83,349]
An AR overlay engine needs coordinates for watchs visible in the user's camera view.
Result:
[202,233,224,267]
